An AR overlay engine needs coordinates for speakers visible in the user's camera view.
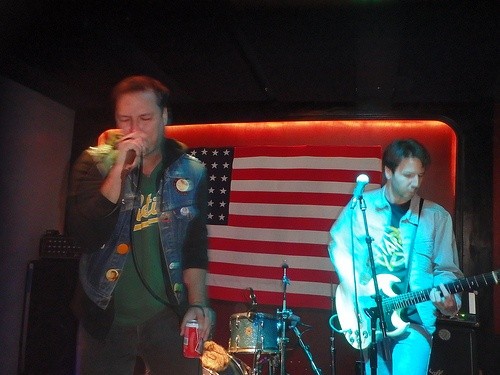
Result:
[426,319,479,375]
[18,259,84,374]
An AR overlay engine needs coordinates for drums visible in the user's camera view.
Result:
[227,311,290,354]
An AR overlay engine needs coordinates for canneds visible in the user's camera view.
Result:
[183,319,202,358]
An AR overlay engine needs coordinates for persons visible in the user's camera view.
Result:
[65,76,215,375]
[328,138,465,375]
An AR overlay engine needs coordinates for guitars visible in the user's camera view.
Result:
[336,269,500,349]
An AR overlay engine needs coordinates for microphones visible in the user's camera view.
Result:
[351,173,370,209]
[125,149,137,170]
[250,288,257,313]
